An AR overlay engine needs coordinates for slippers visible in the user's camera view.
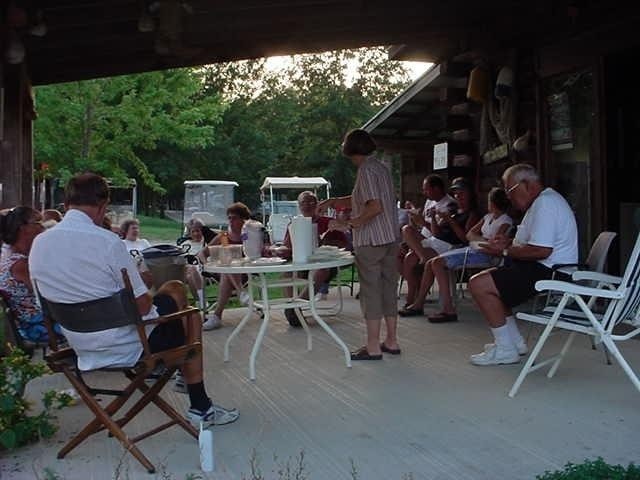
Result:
[351,344,382,360]
[381,343,401,355]
[398,303,424,316]
[428,312,457,323]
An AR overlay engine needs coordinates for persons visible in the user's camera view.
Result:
[100,191,347,391]
[319,129,401,361]
[1,203,63,340]
[28,174,241,431]
[470,163,580,367]
[392,170,515,322]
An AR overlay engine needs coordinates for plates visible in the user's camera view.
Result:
[252,259,287,266]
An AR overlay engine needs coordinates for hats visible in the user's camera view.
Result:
[447,176,465,190]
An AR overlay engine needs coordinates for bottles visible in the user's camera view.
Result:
[262,231,272,258]
[198,430,214,472]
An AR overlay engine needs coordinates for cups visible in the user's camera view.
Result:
[208,243,243,266]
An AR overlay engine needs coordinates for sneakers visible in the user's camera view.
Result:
[239,292,250,305]
[202,314,223,329]
[285,308,306,326]
[186,398,239,431]
[470,337,527,366]
[174,372,187,393]
[145,365,176,382]
